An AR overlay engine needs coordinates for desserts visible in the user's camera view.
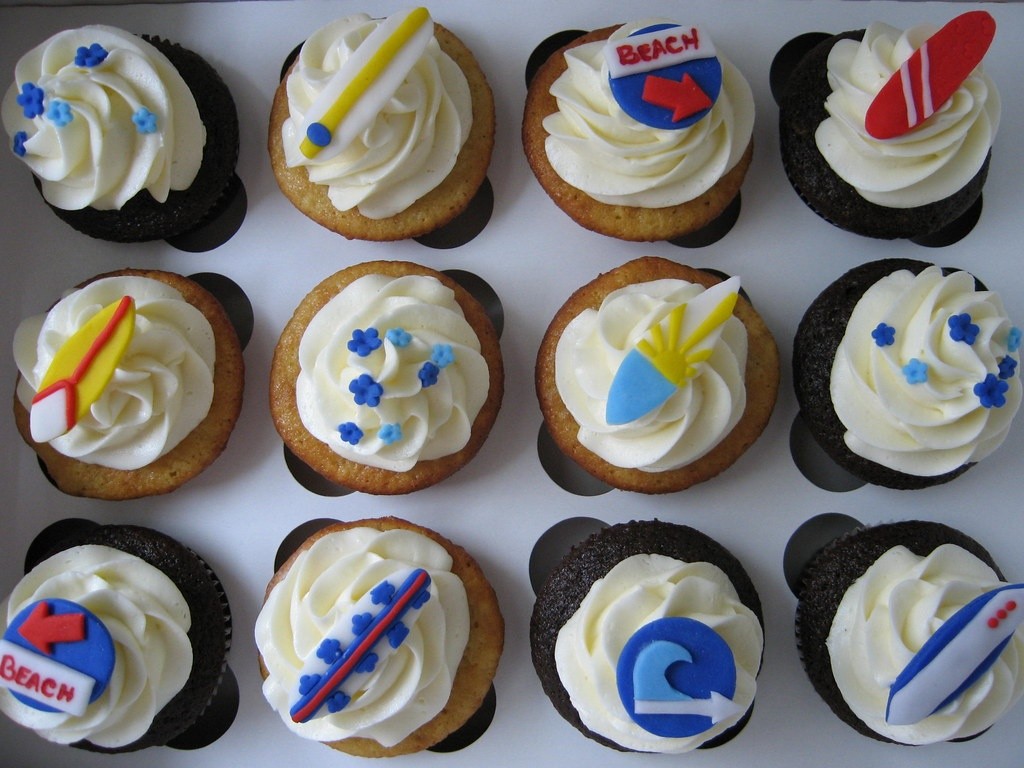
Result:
[2,12,1023,758]
